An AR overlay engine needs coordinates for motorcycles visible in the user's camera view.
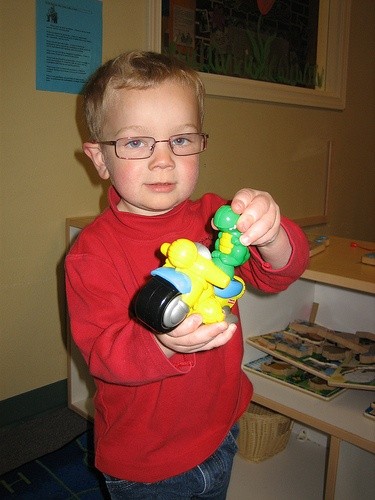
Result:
[128,238,247,336]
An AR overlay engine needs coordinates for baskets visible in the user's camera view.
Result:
[237,401,291,463]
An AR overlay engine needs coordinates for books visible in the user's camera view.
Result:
[361,252,375,266]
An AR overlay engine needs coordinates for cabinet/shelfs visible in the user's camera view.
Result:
[65,215,374,500]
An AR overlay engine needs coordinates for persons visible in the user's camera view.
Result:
[65,49,308,500]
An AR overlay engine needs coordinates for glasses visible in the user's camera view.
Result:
[89,133,209,160]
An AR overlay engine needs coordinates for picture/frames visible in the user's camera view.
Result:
[147,0,351,111]
